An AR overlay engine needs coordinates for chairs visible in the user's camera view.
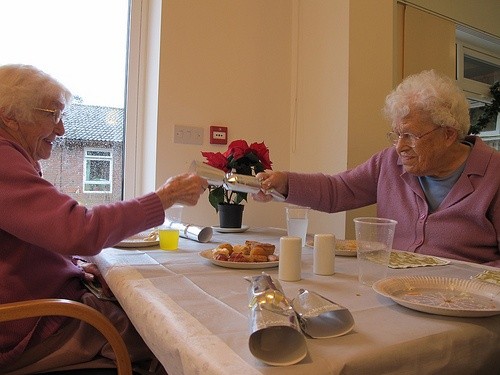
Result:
[0,298,130,375]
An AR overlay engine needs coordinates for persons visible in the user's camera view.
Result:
[251,69,500,267]
[0,64,209,375]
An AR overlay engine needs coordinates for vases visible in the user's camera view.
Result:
[218,202,244,229]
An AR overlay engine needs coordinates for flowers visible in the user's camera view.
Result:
[200,138,274,214]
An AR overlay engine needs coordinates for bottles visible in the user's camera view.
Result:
[312,233,336,275]
[278,236,302,281]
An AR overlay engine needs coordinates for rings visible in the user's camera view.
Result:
[202,186,206,191]
[258,177,265,181]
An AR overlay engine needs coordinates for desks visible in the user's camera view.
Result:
[93,228,500,375]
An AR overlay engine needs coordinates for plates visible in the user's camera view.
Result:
[306,239,386,257]
[113,230,160,248]
[372,275,500,318]
[198,246,279,268]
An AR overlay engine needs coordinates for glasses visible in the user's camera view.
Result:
[384,126,441,147]
[33,106,66,124]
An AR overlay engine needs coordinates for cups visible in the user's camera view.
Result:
[284,206,311,247]
[353,216,398,269]
[159,204,184,252]
